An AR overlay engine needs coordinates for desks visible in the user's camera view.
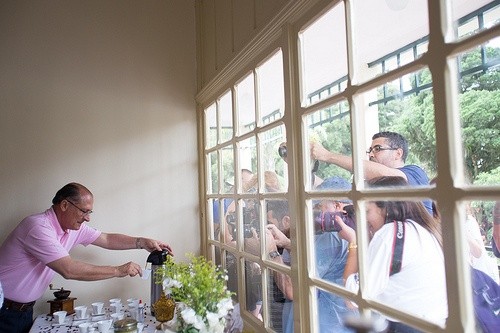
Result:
[29,304,164,333]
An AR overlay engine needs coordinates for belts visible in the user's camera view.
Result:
[3,298,36,311]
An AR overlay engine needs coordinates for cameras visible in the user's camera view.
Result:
[322,205,357,232]
[226,207,250,225]
[279,145,288,158]
[232,218,260,238]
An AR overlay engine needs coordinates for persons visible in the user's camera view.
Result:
[211,131,500,333]
[0,182,174,333]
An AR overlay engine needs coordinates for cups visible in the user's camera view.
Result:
[73,306,87,318]
[91,302,105,314]
[53,310,67,324]
[139,269,152,280]
[109,297,146,333]
[78,318,113,333]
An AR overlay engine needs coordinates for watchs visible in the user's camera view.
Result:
[268,251,280,260]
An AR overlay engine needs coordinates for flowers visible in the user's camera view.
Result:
[153,251,238,319]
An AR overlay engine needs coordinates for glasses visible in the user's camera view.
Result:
[366,146,399,155]
[66,200,93,216]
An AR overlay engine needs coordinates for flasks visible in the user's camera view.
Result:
[147,248,175,317]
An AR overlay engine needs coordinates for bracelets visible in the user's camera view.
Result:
[349,243,357,248]
[136,238,142,248]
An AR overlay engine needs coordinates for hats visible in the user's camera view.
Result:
[225,176,235,187]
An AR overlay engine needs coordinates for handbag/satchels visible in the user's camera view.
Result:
[471,266,500,333]
